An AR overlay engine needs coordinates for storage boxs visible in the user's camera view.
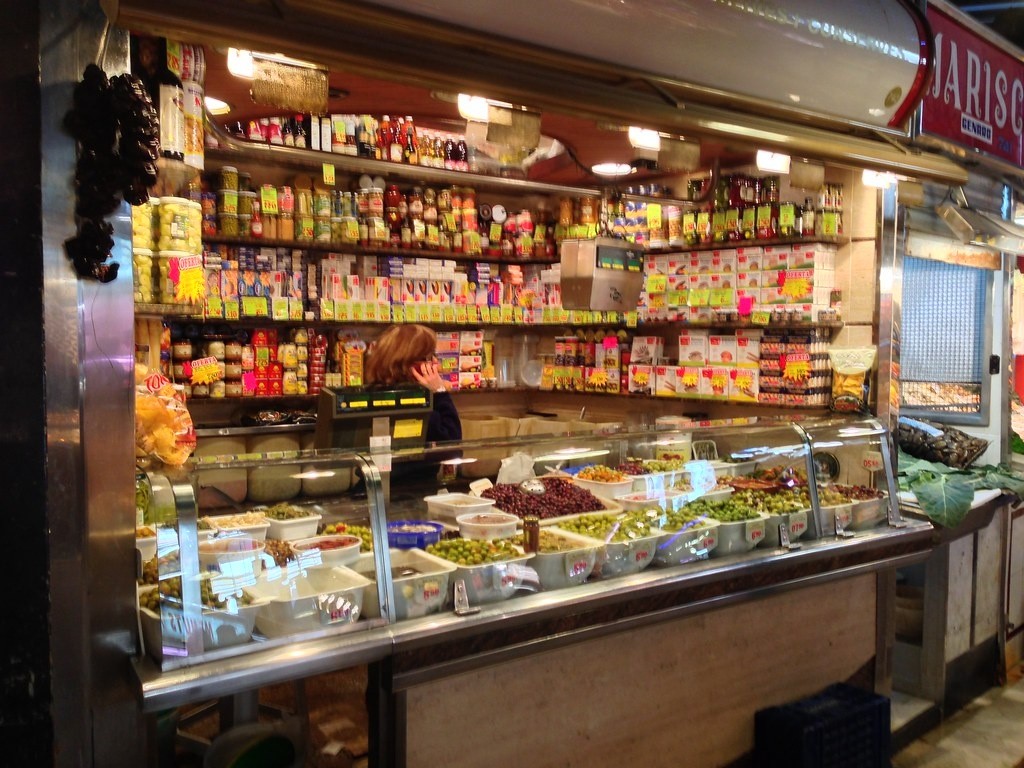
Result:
[201,241,836,478]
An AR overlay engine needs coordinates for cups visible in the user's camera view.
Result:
[495,333,545,388]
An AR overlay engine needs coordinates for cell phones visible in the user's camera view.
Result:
[407,361,438,385]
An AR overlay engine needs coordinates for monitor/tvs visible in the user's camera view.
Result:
[313,383,434,494]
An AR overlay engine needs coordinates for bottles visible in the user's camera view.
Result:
[523,516,539,553]
[250,202,263,238]
[206,110,470,173]
[555,171,846,248]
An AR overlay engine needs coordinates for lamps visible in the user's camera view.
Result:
[626,126,700,172]
[861,168,924,206]
[754,149,826,192]
[225,46,330,119]
[455,94,542,150]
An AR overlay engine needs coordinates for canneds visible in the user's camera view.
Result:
[203,167,558,261]
[169,324,328,397]
[553,336,596,391]
[559,172,805,243]
[814,181,844,236]
[523,515,540,552]
[131,42,205,388]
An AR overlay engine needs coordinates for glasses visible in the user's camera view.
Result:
[412,350,437,364]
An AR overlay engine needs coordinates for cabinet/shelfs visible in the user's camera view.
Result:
[183,105,848,418]
[890,487,1018,724]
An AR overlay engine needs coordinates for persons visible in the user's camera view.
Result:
[365,323,464,489]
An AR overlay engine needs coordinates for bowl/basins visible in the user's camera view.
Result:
[455,512,520,538]
[385,519,444,548]
[198,537,266,573]
[289,535,364,564]
